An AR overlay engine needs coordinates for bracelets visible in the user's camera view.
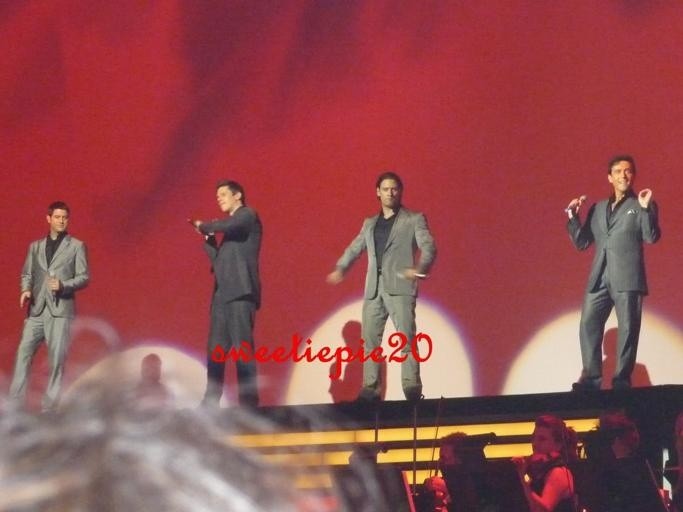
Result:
[641,206,651,212]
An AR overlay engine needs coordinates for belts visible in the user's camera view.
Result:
[378,271,382,275]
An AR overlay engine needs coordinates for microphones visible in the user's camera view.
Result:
[567,195,587,210]
[49,270,57,303]
[395,271,427,279]
[187,218,216,242]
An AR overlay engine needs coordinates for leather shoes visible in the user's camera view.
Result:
[611,372,631,388]
[572,379,602,392]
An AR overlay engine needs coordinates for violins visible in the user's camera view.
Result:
[509,450,560,464]
[432,463,446,511]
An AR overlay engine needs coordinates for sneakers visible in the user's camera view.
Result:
[340,392,382,404]
[407,393,424,400]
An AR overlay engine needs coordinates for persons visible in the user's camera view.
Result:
[188,180,262,409]
[565,155,660,391]
[342,414,679,512]
[8,202,90,413]
[326,171,436,401]
